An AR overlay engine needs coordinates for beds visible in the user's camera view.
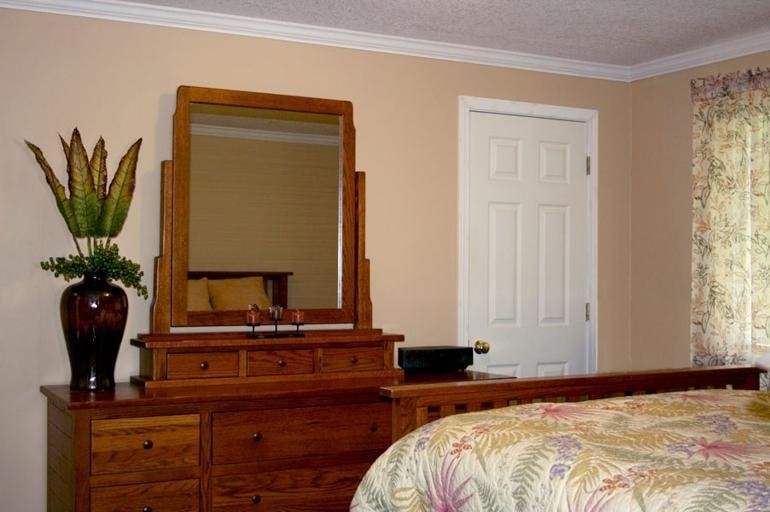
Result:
[349,367,770,512]
[187,271,294,312]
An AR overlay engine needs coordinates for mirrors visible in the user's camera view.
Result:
[170,85,357,327]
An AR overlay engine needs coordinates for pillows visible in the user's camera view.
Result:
[186,276,212,311]
[208,276,272,310]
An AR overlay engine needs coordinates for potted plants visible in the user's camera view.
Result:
[23,125,148,392]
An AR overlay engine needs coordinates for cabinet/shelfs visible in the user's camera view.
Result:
[89,412,203,512]
[210,401,392,512]
[129,332,404,388]
